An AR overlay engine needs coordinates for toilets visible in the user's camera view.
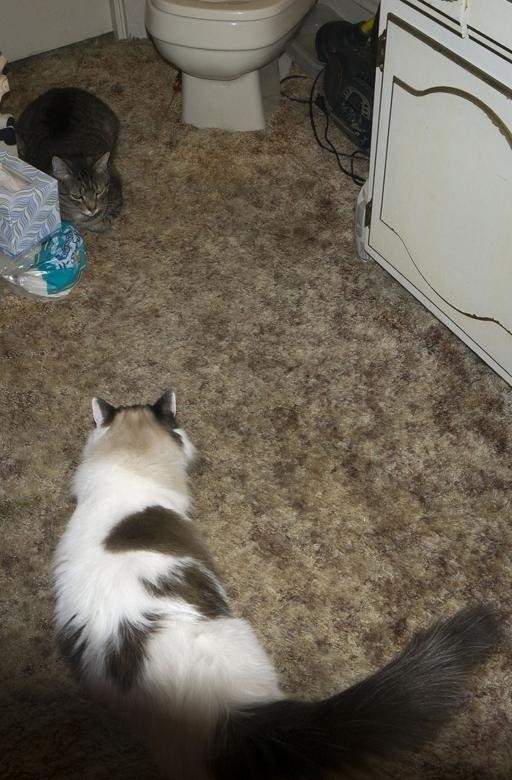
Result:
[144,0,314,132]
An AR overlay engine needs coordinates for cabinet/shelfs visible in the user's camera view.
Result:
[356,1,510,380]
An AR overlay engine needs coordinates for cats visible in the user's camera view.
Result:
[13,86,124,234]
[49,390,511,780]
